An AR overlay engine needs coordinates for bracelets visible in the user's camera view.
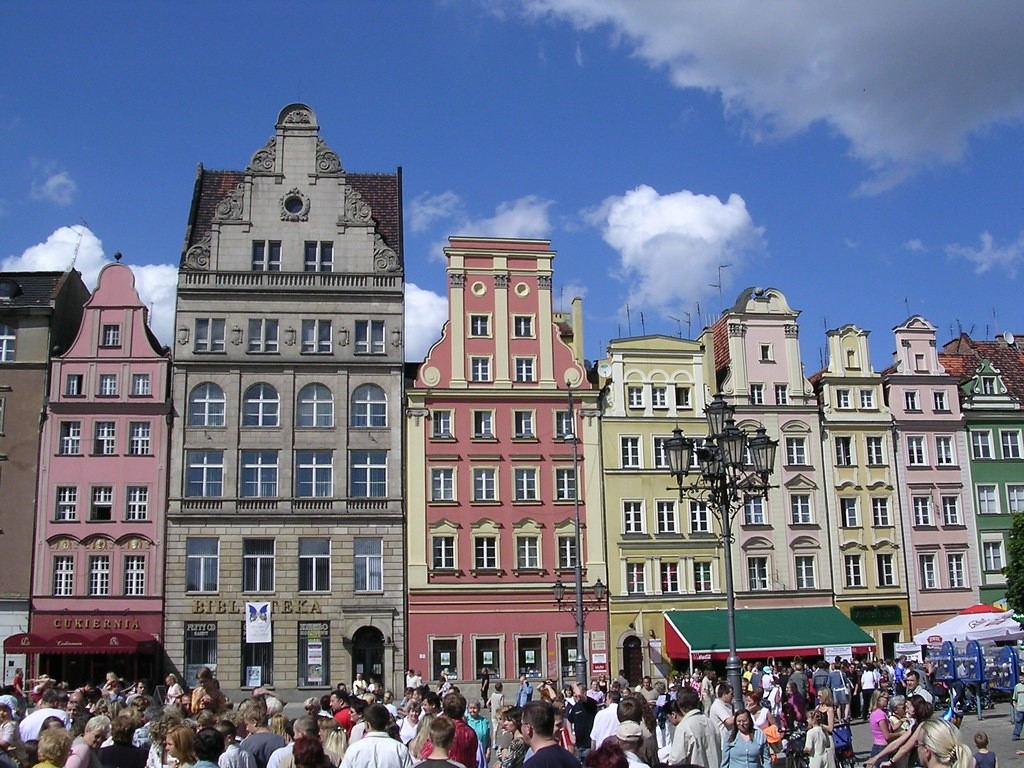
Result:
[890,758,895,765]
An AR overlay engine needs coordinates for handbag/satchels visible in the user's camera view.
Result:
[845,687,851,695]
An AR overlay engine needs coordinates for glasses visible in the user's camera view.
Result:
[92,733,107,742]
[351,711,357,713]
[914,739,926,750]
[881,696,890,701]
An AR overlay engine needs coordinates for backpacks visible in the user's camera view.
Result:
[760,690,771,713]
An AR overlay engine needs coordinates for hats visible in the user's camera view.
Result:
[763,666,772,674]
[614,720,642,743]
[0,694,20,720]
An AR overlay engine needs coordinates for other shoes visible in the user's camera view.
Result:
[844,718,849,723]
[848,716,853,721]
[492,745,498,750]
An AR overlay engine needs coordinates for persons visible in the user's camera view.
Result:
[0,655,998,768]
[1012,672,1024,741]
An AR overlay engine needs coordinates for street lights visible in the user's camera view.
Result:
[661,392,781,716]
[551,433,607,691]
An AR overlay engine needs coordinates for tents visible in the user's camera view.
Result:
[913,603,1024,646]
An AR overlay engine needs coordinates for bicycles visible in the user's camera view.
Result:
[777,718,859,768]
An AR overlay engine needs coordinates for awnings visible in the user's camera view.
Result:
[664,607,877,675]
[2,632,157,694]
